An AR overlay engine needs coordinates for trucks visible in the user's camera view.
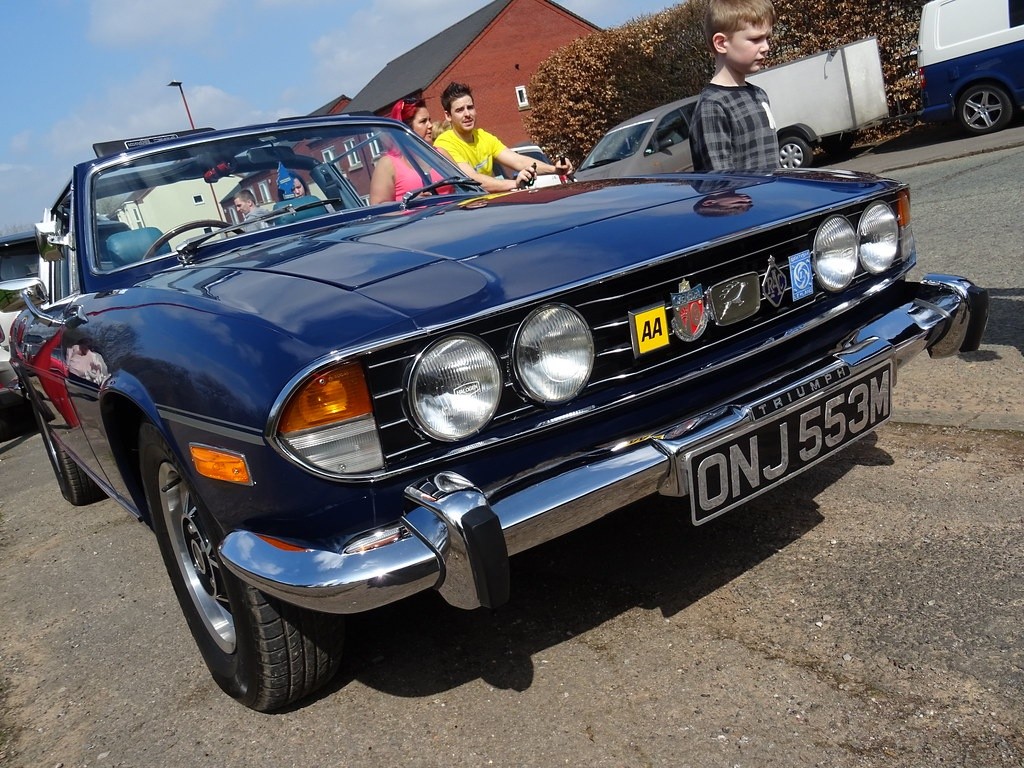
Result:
[740,37,890,169]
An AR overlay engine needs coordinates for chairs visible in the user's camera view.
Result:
[273,195,327,226]
[105,227,172,268]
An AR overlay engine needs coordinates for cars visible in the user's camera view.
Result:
[10,110,990,714]
[572,95,699,181]
[0,217,132,439]
[491,144,567,189]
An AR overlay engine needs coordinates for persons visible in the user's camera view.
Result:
[433,82,573,194]
[277,172,329,214]
[689,179,754,217]
[370,98,459,216]
[234,189,275,233]
[688,0,779,171]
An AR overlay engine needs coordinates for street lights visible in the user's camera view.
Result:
[167,80,195,129]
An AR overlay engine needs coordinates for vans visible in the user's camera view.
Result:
[917,0,1024,135]
